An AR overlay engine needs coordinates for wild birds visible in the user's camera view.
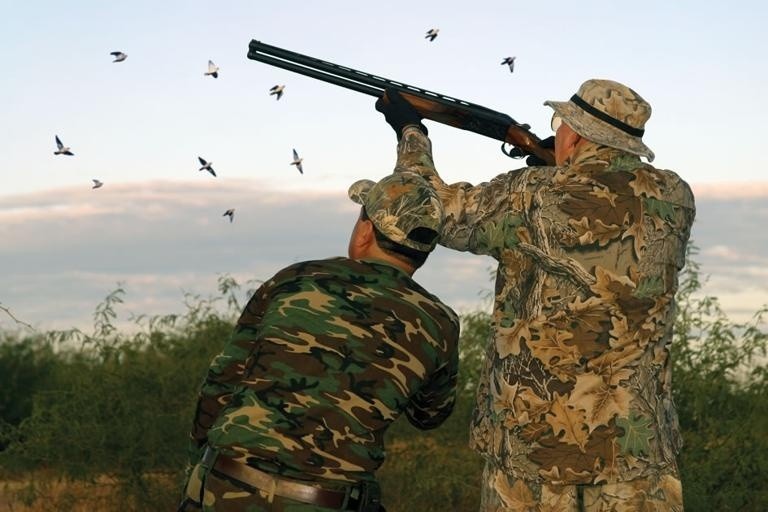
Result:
[222,207,235,222]
[268,83,286,101]
[424,27,440,42]
[197,156,217,178]
[92,178,103,190]
[204,60,221,79]
[500,56,516,73]
[290,148,304,175]
[54,134,74,156]
[109,51,128,64]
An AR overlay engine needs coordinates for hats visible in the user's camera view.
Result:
[543,79,655,164]
[348,172,445,252]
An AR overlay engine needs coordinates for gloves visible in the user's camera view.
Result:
[374,88,429,138]
[508,136,558,166]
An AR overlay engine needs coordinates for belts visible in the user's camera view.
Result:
[201,446,360,509]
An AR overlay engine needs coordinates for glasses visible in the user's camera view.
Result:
[550,112,585,148]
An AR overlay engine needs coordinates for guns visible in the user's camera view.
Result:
[247,39,555,167]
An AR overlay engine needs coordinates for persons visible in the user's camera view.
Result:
[179,170,460,510]
[372,74,697,510]
